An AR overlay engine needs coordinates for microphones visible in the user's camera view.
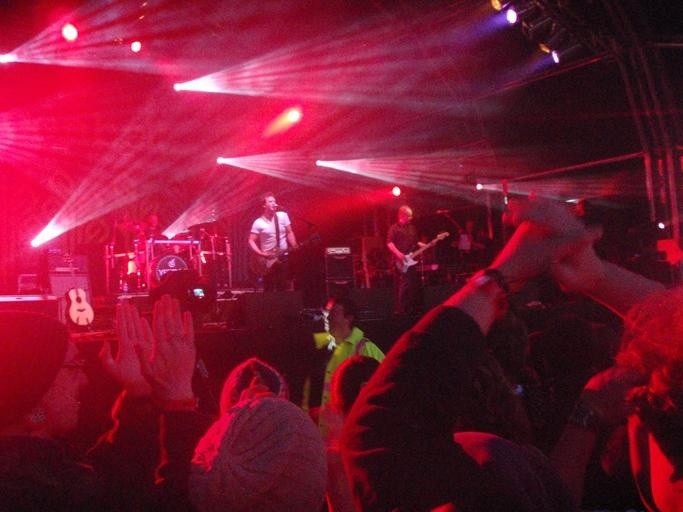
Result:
[274,203,286,211]
[436,210,448,215]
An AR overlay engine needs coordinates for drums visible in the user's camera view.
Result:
[143,253,193,289]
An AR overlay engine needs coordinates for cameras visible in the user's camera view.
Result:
[505,379,528,399]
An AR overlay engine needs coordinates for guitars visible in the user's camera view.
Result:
[395,232,450,273]
[65,253,95,327]
[250,230,321,277]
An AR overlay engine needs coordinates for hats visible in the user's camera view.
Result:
[189,397,327,512]
[0,310,68,428]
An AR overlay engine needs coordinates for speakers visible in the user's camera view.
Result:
[405,286,451,313]
[231,291,304,329]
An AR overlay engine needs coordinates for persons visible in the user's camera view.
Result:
[1,193,681,510]
